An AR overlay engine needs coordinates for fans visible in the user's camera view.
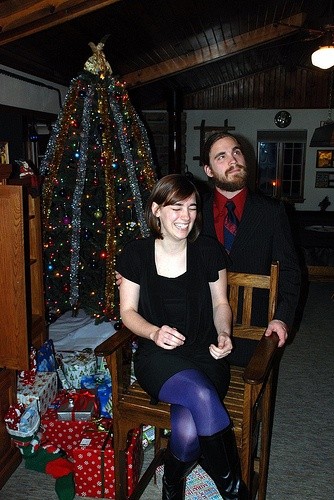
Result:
[275,15,334,56]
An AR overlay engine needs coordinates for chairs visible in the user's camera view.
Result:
[93,258,280,500]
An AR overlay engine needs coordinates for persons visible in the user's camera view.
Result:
[116,174,249,500]
[115,132,302,366]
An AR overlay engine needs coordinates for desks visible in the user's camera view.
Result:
[304,225,334,266]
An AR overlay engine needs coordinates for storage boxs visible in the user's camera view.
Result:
[4,340,227,500]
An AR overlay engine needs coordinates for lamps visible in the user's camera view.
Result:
[310,41,334,70]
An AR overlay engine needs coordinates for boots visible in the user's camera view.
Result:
[198,419,253,500]
[162,437,200,500]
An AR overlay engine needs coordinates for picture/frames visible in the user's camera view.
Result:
[316,149,334,169]
[315,171,334,188]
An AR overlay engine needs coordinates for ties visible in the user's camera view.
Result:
[223,199,239,254]
